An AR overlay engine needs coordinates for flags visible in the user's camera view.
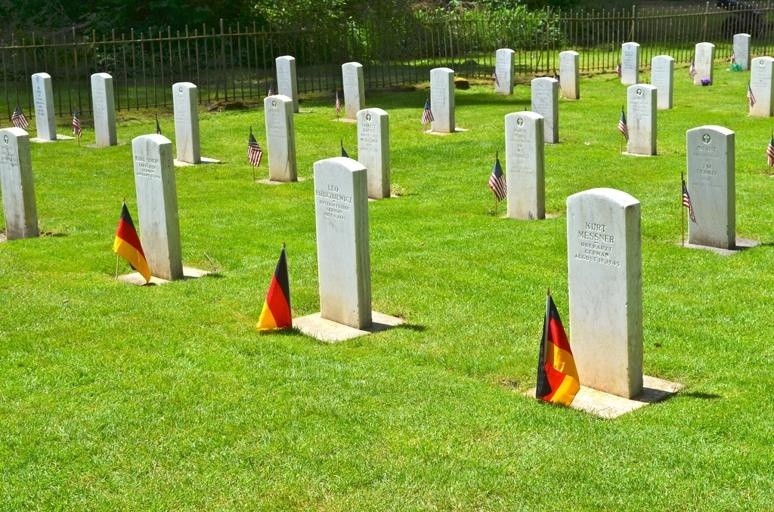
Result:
[535,289,580,409]
[765,135,774,168]
[615,63,620,77]
[746,87,757,106]
[268,85,273,97]
[155,119,161,133]
[730,51,737,65]
[682,179,695,223]
[11,100,29,130]
[256,242,295,332]
[338,142,349,157]
[617,106,629,141]
[421,98,435,126]
[247,129,263,167]
[113,200,153,285]
[70,112,83,141]
[334,95,341,113]
[553,75,559,86]
[688,63,698,79]
[488,150,507,202]
[495,74,500,87]
